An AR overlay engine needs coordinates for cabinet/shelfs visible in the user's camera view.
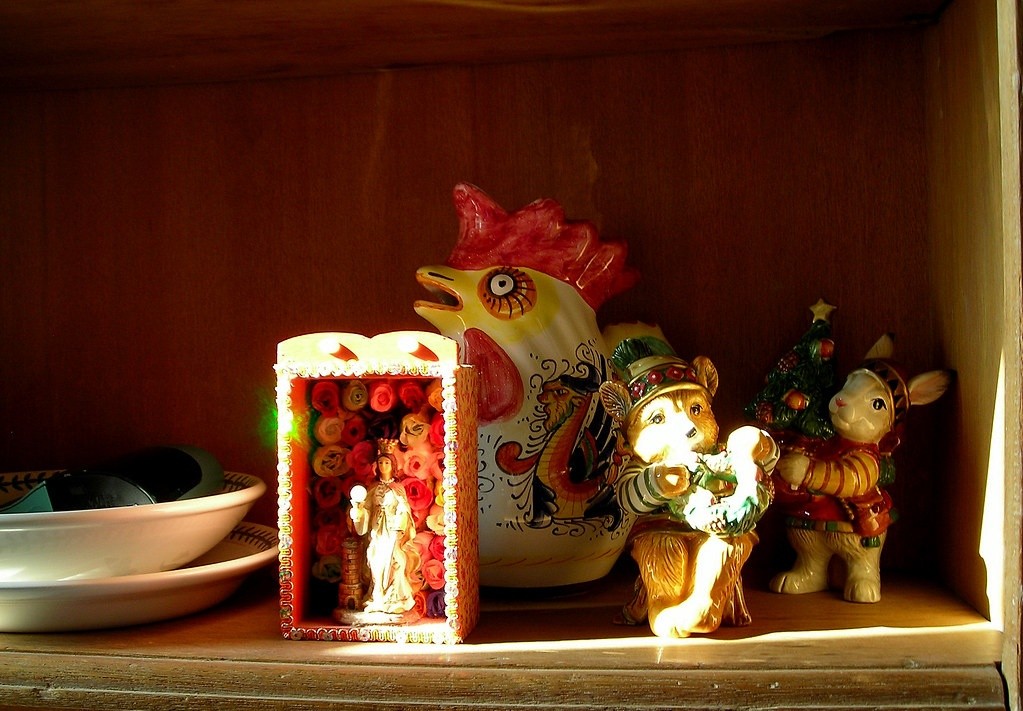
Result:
[0,0,1023,711]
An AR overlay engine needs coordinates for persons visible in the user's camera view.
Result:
[351,451,417,611]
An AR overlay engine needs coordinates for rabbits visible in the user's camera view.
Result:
[767,332,960,603]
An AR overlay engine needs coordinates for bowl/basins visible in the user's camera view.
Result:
[0,520,281,632]
[0,468,268,583]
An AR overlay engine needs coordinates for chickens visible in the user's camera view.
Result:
[412,180,672,587]
